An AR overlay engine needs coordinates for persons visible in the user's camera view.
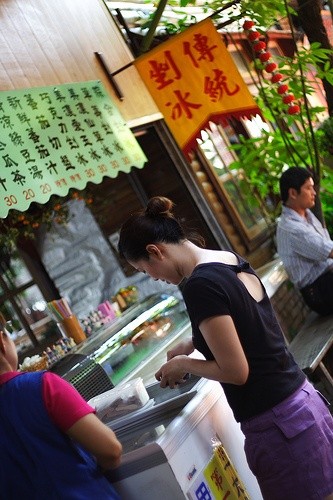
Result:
[118,197,333,500]
[42,337,77,365]
[0,311,123,500]
[276,167,333,318]
[82,310,107,336]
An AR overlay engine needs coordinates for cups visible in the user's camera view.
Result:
[56,314,87,345]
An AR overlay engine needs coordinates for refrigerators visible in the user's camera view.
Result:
[43,291,194,409]
[100,373,264,500]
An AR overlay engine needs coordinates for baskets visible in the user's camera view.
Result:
[24,356,47,371]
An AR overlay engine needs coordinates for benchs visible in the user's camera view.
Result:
[287,311,333,418]
